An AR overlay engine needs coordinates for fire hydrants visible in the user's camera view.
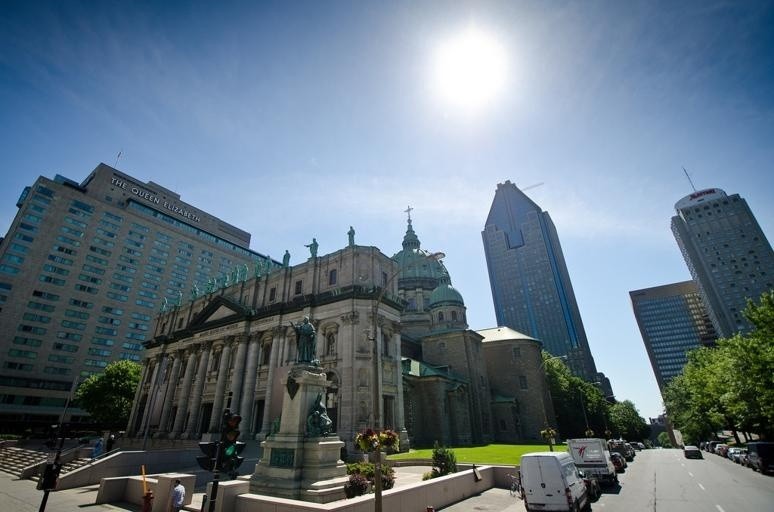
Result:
[141,489,154,512]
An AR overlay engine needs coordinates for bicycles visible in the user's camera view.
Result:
[504,470,523,499]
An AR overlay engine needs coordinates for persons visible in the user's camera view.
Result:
[307,390,332,437]
[91,434,115,461]
[305,238,319,260]
[347,226,355,245]
[283,250,291,267]
[176,290,183,307]
[290,316,318,365]
[159,297,167,311]
[171,479,186,512]
[191,256,273,298]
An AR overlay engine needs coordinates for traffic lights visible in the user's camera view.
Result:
[220,414,241,471]
[43,476,56,490]
[234,442,246,468]
[196,440,215,469]
[42,423,58,448]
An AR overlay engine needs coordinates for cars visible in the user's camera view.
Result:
[578,471,601,499]
[70,431,125,446]
[607,438,646,471]
[700,440,774,475]
[684,445,701,458]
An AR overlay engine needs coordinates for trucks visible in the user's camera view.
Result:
[567,437,624,485]
[520,452,590,512]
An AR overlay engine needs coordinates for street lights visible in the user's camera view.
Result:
[532,354,568,451]
[374,251,446,512]
[579,380,602,429]
[601,394,617,429]
